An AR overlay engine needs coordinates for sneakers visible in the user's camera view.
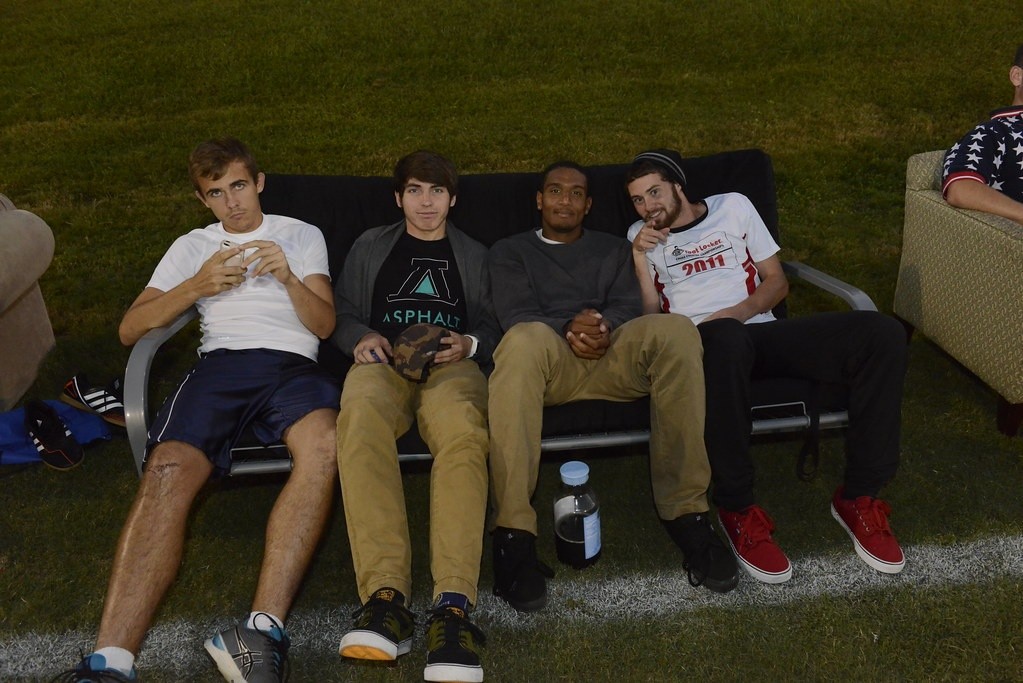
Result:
[51,646,135,683]
[717,504,793,584]
[424,604,487,683]
[339,587,415,660]
[664,511,740,593]
[831,484,906,574]
[491,528,555,611]
[204,612,291,682]
[59,374,126,426]
[24,395,84,470]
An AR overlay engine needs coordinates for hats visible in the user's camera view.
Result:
[393,324,453,379]
[634,147,687,186]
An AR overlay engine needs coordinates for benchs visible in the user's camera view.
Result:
[122,149,877,479]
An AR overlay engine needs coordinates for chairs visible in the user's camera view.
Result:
[892,151,1023,439]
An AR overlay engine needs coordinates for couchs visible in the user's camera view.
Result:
[0,193,57,416]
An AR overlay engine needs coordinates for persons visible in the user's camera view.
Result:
[464,334,481,358]
[942,48,1023,225]
[333,149,499,683]
[58,139,339,682]
[627,149,905,583]
[488,161,739,612]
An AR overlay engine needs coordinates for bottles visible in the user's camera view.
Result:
[554,460,602,569]
